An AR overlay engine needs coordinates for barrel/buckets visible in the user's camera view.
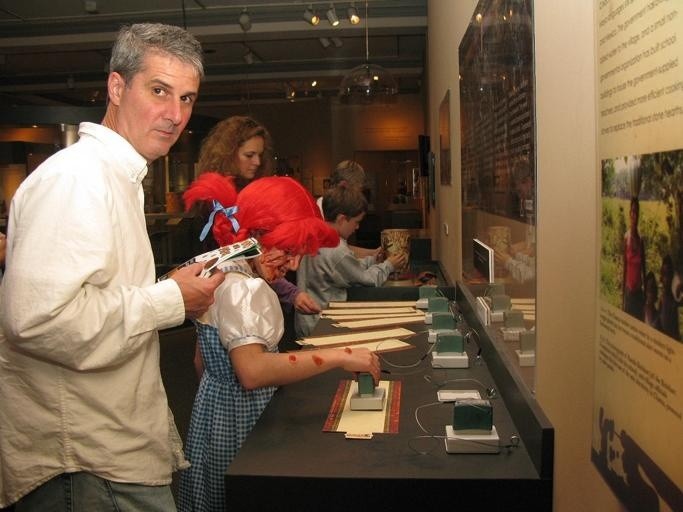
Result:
[380,230,412,274]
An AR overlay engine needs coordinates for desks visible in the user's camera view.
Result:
[223,300,554,511]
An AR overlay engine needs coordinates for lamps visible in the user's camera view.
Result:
[238,0,399,102]
[66,76,75,88]
[87,88,102,105]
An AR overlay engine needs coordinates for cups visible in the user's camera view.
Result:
[487,227,511,277]
[380,229,411,282]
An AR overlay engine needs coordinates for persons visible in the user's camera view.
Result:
[316,160,367,229]
[489,249,535,284]
[1,21,225,512]
[623,196,648,320]
[642,270,659,326]
[171,115,321,320]
[517,156,535,255]
[655,253,680,340]
[288,182,406,338]
[170,176,381,512]
